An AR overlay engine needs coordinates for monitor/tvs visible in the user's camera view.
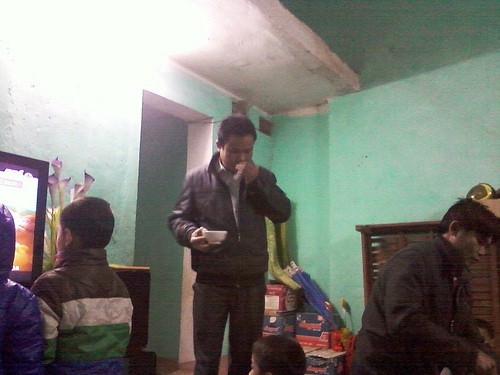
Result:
[0,151,49,288]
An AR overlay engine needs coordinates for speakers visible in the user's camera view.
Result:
[112,266,150,350]
[124,351,156,375]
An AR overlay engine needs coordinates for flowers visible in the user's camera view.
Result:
[42,157,95,272]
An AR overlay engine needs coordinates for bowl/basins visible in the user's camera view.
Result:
[202,230,228,245]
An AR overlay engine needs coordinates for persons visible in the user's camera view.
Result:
[29,196,134,375]
[350,197,500,375]
[0,201,47,375]
[248,334,306,375]
[167,112,291,375]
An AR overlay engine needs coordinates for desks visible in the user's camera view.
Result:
[109,264,156,375]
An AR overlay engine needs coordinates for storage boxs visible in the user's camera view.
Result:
[262,284,347,375]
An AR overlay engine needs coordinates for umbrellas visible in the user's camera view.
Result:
[290,260,339,330]
